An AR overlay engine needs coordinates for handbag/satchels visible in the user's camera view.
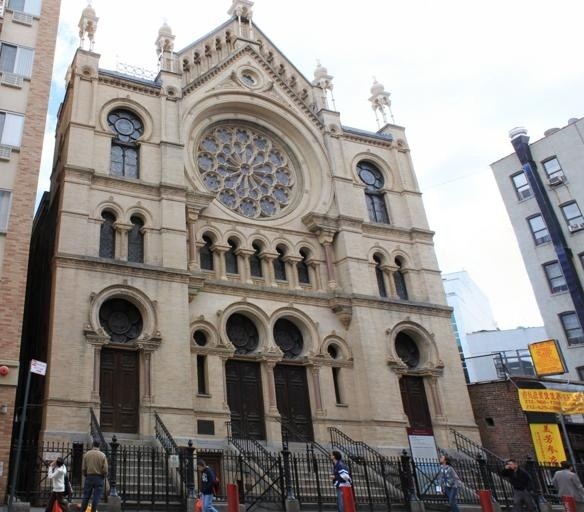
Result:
[65,479,74,496]
[211,476,221,496]
[196,497,203,512]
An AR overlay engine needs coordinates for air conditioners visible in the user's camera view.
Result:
[547,176,563,186]
[567,223,584,232]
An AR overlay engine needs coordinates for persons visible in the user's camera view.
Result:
[501,460,538,512]
[45,457,69,512]
[197,459,220,512]
[329,451,353,512]
[552,461,584,512]
[439,455,462,512]
[79,441,109,512]
